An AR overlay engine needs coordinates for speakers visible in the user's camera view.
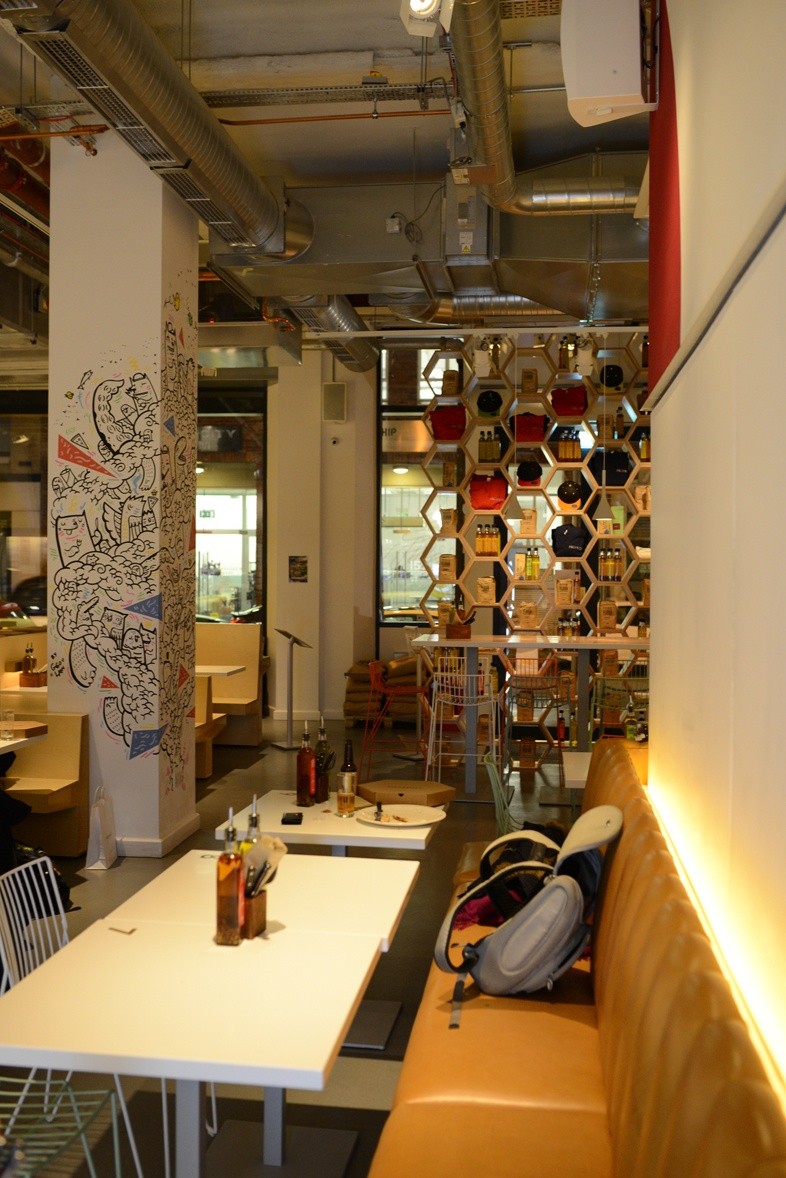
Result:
[559,0,661,127]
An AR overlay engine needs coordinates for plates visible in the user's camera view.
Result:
[354,804,447,828]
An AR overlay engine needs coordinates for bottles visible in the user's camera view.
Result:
[333,738,358,819]
[296,731,316,808]
[22,647,36,674]
[426,334,649,640]
[559,709,565,742]
[316,725,327,804]
[219,825,243,947]
[569,712,578,748]
[239,812,268,940]
[624,704,638,739]
[636,710,647,744]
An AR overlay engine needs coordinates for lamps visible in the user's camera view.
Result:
[397,0,455,38]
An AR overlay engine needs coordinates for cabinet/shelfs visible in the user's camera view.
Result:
[413,334,654,767]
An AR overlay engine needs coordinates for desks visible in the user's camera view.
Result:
[103,847,423,1178]
[214,787,450,1051]
[1,918,386,1178]
[410,633,650,807]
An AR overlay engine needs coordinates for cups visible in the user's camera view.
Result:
[0,709,15,741]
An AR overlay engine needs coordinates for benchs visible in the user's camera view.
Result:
[366,737,786,1177]
[1,619,264,858]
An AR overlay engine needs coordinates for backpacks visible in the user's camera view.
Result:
[432,805,623,995]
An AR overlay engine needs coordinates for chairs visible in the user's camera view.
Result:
[0,1070,138,1177]
[0,855,222,1140]
[360,655,649,796]
